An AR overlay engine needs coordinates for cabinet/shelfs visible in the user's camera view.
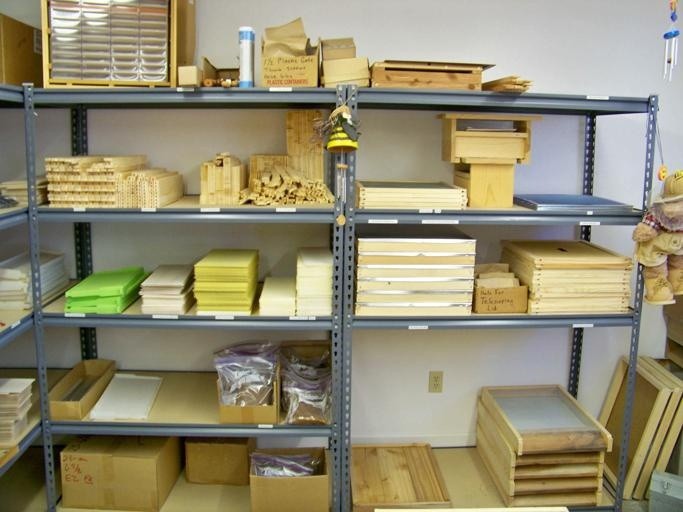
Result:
[0,82,658,512]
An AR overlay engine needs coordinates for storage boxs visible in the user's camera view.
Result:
[249,447,330,512]
[0,13,43,87]
[59,436,181,512]
[185,437,257,486]
